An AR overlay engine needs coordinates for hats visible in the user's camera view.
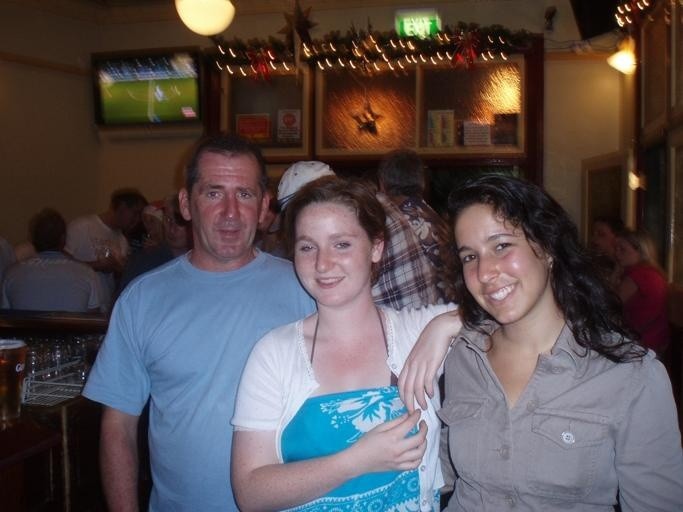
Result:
[141,200,165,223]
[267,162,336,234]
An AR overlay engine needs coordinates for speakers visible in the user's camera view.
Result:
[570,0,626,39]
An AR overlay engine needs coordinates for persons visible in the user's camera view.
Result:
[279,160,435,311]
[377,148,454,305]
[81,132,318,512]
[0,187,193,336]
[436,175,682,511]
[230,174,466,512]
[592,208,673,356]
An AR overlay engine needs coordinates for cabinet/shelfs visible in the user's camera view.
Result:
[223,63,310,211]
[419,58,543,229]
[635,20,683,284]
[311,61,419,156]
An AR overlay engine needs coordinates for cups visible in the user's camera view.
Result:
[27,335,97,380]
[0,339,27,431]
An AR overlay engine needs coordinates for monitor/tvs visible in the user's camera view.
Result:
[90,45,205,128]
